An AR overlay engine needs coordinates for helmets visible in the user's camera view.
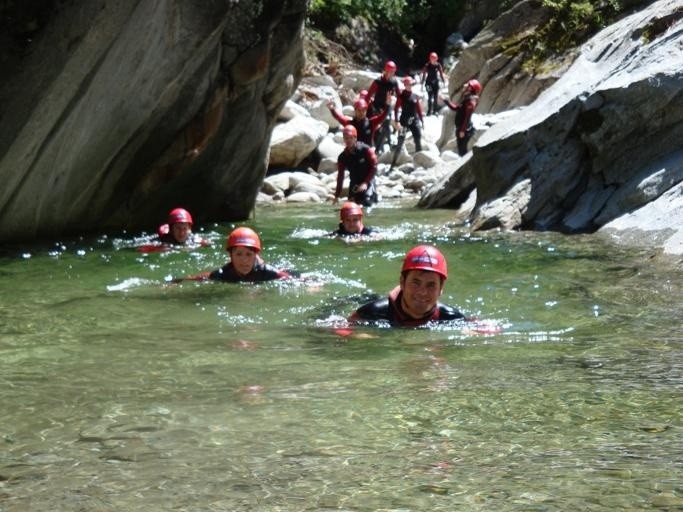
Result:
[429,51,438,63]
[360,88,369,99]
[343,124,358,140]
[468,78,482,95]
[157,225,170,238]
[168,208,193,230]
[401,246,448,279]
[227,227,261,252]
[404,75,417,87]
[354,101,369,110]
[341,201,364,218]
[385,60,398,74]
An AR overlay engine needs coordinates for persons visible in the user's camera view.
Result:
[364,61,401,153]
[208,227,292,285]
[387,76,425,175]
[153,209,206,246]
[344,244,464,330]
[333,201,370,237]
[359,90,384,155]
[439,78,481,156]
[333,125,379,207]
[421,53,446,117]
[325,91,392,149]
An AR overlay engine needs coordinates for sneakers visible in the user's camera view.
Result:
[385,168,393,177]
